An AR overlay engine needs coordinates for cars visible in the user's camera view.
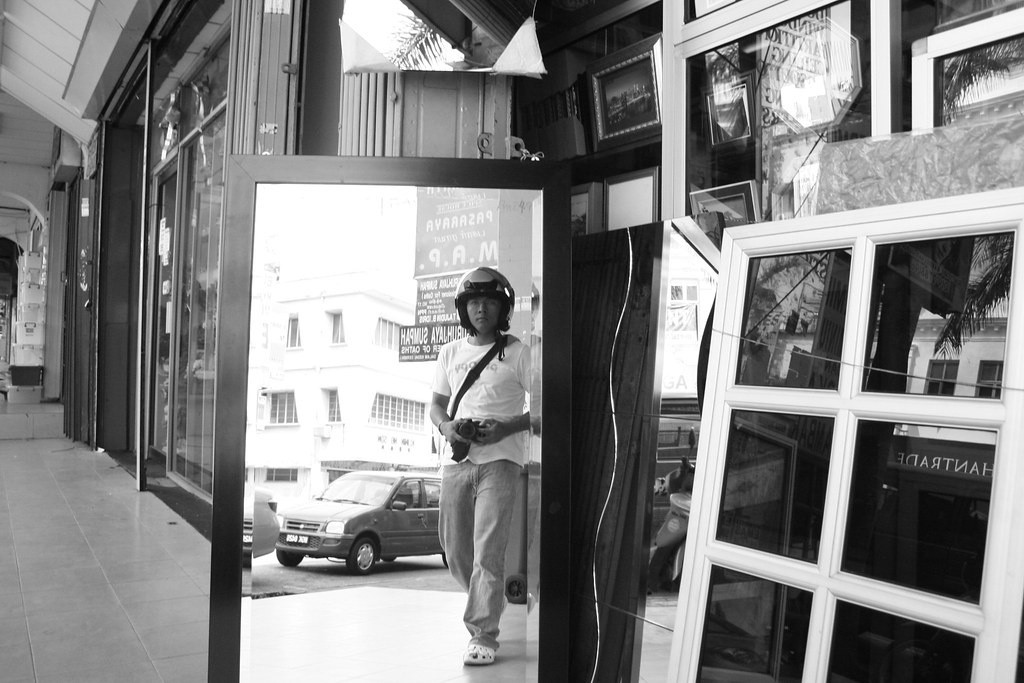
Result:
[244,482,280,561]
[276,469,451,576]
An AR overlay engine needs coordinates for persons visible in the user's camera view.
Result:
[430,267,532,665]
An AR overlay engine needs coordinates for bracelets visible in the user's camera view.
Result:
[438,420,445,435]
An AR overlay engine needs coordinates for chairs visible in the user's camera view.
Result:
[397,488,414,509]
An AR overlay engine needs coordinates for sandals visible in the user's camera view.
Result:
[464,641,495,665]
[500,595,508,611]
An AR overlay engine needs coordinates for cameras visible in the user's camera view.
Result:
[456,420,487,441]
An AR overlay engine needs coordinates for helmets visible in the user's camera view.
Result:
[455,267,515,331]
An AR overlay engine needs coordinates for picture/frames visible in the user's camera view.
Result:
[586,31,662,153]
[701,69,758,152]
[603,165,660,232]
[690,180,761,227]
[701,416,797,683]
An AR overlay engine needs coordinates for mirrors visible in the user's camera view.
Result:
[207,152,574,683]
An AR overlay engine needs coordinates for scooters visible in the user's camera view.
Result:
[645,428,698,594]
